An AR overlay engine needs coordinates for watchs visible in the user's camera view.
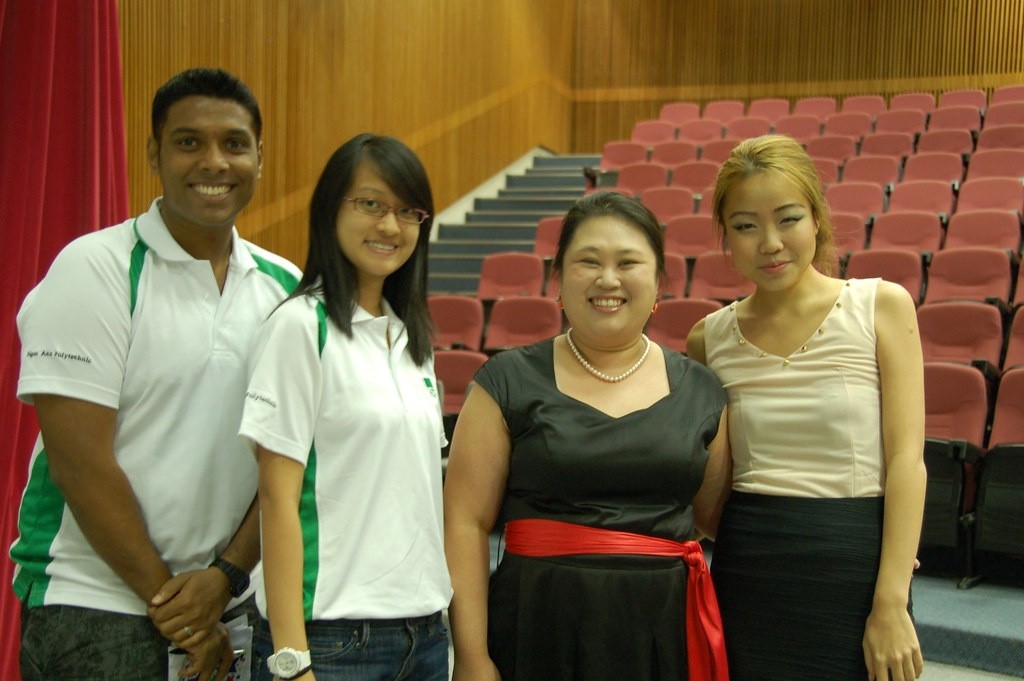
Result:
[209,558,250,598]
[266,647,310,679]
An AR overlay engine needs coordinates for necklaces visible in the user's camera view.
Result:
[567,328,651,382]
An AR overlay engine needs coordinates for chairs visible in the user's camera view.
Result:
[425,85,1024,591]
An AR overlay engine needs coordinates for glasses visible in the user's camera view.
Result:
[341,196,429,225]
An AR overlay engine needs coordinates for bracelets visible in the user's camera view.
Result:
[280,664,313,681]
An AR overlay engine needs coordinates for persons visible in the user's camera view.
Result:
[441,193,920,681]
[240,132,455,681]
[9,69,302,681]
[683,134,928,681]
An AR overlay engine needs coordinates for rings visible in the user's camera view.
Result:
[185,626,193,635]
